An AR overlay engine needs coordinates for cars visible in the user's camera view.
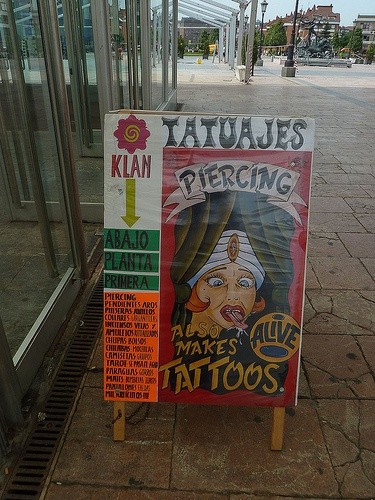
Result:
[349,54,366,64]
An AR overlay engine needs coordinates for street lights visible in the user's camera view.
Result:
[244,15,249,51]
[254,0,268,66]
